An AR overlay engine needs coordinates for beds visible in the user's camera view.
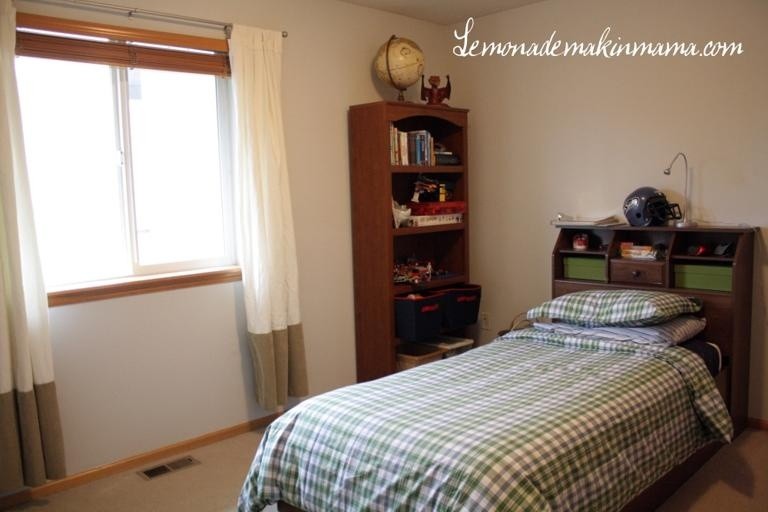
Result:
[237,331,734,512]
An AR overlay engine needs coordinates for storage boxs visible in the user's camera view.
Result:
[557,255,606,282]
[674,261,734,294]
[393,284,480,372]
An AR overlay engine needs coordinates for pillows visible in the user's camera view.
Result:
[525,289,704,349]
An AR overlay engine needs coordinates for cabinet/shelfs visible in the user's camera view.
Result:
[348,102,470,383]
[547,220,755,333]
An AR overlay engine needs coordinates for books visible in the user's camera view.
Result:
[390,124,436,168]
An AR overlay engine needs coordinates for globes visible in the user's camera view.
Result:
[374,35,425,101]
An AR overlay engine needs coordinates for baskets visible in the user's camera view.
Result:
[396,334,475,372]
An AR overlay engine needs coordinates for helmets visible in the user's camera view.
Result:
[623,186,666,227]
